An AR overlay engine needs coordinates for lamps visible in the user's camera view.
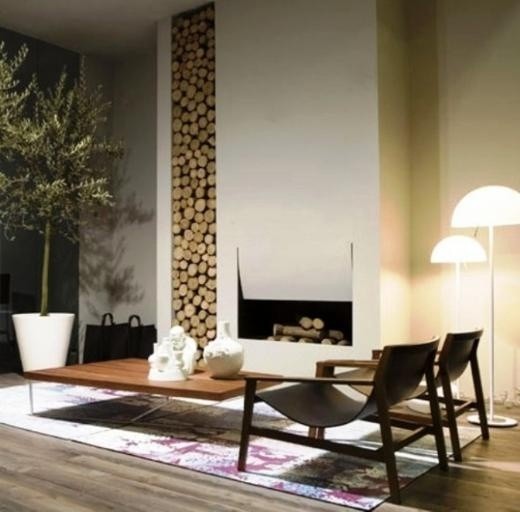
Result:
[429,234,489,407]
[449,181,520,428]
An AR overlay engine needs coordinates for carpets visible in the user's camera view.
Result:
[0,381,486,511]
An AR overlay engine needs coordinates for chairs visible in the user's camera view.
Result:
[372,329,490,463]
[238,336,450,505]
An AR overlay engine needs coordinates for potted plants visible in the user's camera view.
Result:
[0,41,129,376]
